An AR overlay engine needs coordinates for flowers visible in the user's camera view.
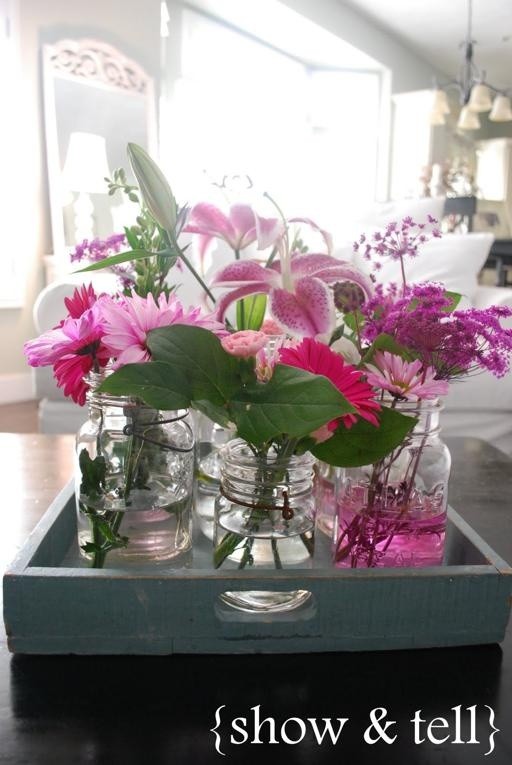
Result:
[22,141,512,571]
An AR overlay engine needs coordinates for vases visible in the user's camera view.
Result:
[73,372,451,614]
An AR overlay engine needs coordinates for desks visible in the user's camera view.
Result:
[0,430,511,765]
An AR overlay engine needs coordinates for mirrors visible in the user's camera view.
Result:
[40,38,158,256]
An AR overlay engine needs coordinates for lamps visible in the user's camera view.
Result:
[427,0,512,130]
[62,132,113,245]
